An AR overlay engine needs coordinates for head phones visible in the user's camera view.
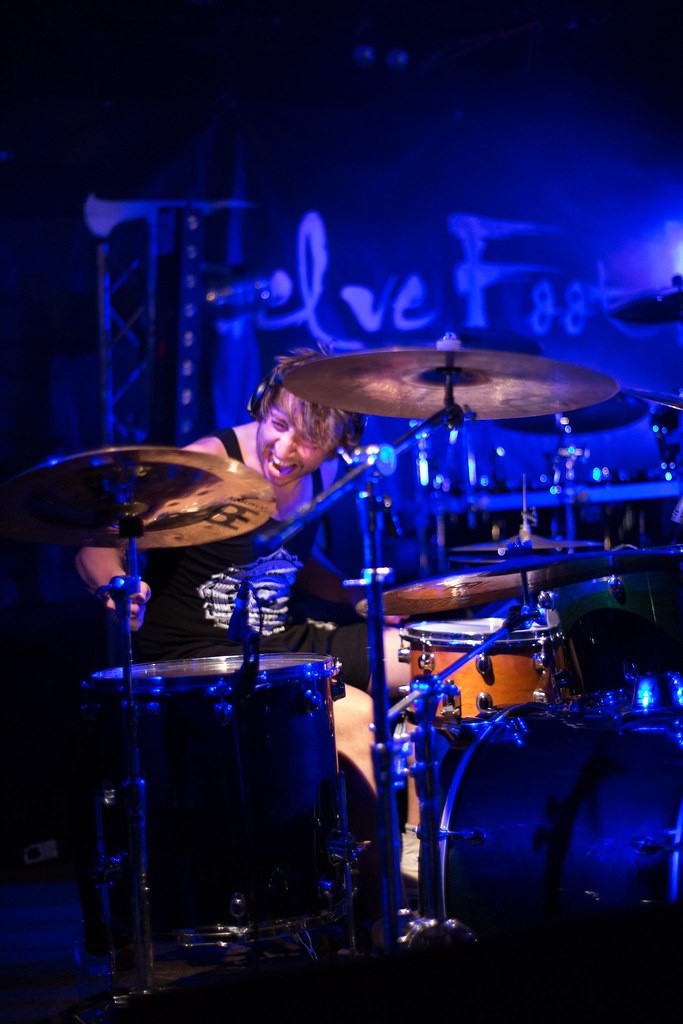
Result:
[247,370,367,458]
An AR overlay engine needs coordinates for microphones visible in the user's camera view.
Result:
[227,583,250,642]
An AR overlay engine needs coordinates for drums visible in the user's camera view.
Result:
[75,648,359,948]
[416,701,683,920]
[398,616,571,728]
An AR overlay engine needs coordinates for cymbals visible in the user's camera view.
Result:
[624,383,682,412]
[495,392,648,432]
[354,545,683,617]
[609,282,683,325]
[277,344,623,421]
[3,444,277,554]
[449,531,604,566]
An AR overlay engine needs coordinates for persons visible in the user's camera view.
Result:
[77,340,458,946]
